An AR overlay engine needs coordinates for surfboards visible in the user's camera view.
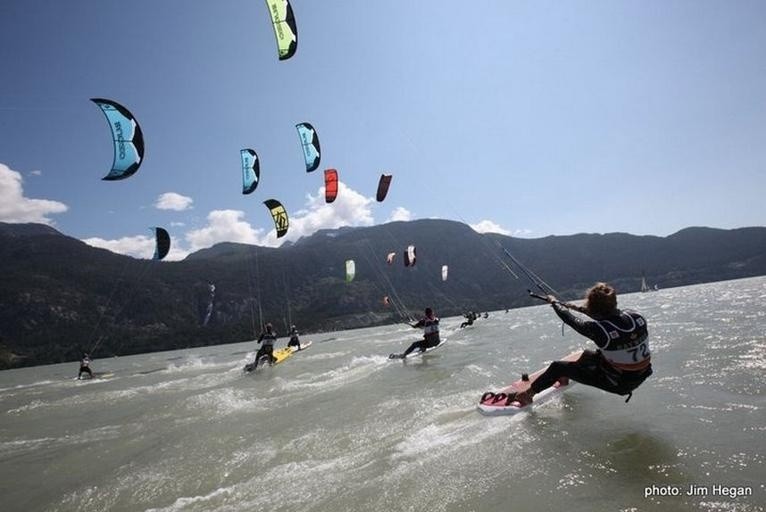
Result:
[386,337,448,362]
[477,349,584,416]
[242,340,312,373]
[74,373,115,379]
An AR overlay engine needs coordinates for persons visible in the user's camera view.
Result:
[391,307,440,358]
[77,353,93,379]
[461,311,488,328]
[508,282,653,406]
[288,325,301,350]
[248,323,277,370]
